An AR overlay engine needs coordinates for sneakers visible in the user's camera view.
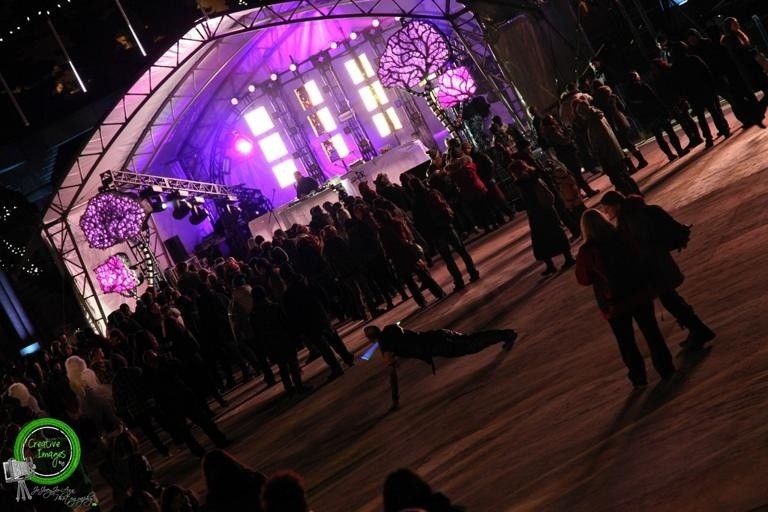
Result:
[675,325,717,355]
[282,383,315,400]
[351,272,493,322]
[665,148,690,161]
[627,158,649,176]
[629,360,679,390]
[150,395,240,466]
[326,353,356,381]
[539,264,559,277]
[501,329,518,352]
[561,258,576,270]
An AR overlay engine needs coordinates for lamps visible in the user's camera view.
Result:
[418,70,439,88]
[229,63,297,106]
[172,199,209,225]
[330,17,382,50]
[153,202,167,212]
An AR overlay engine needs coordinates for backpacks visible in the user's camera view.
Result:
[534,177,556,211]
[423,186,455,228]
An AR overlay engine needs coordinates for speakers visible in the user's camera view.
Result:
[163,235,190,265]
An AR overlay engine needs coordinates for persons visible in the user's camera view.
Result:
[597,190,717,378]
[570,207,673,391]
[3,244,469,510]
[234,1,768,315]
[363,321,518,412]
